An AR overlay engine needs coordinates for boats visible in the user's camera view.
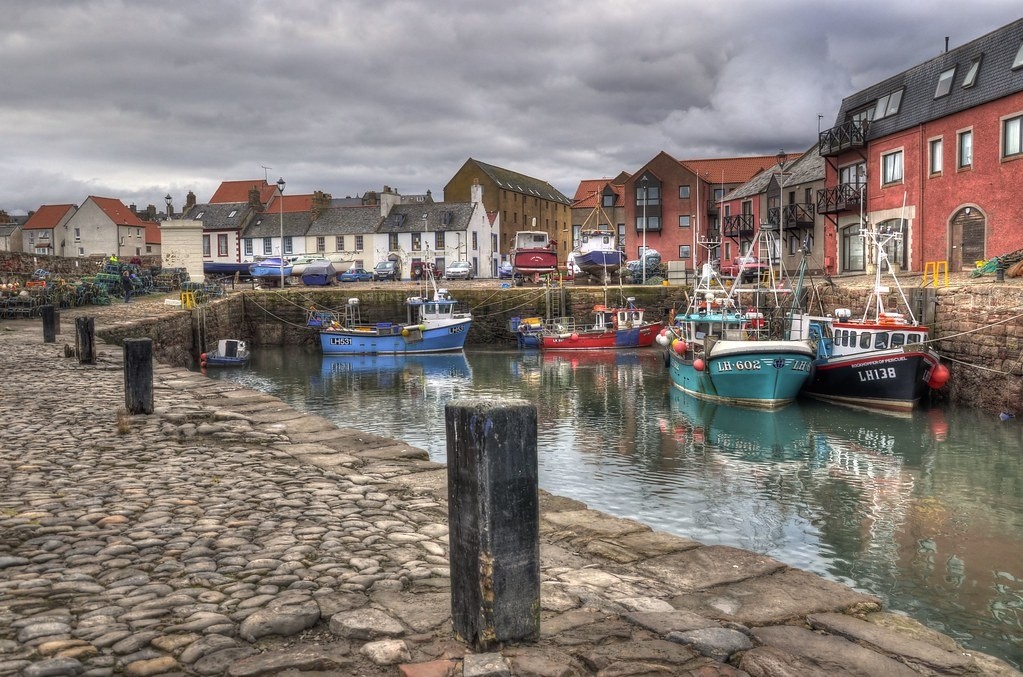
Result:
[203,256,298,280]
[300,258,337,287]
[292,254,356,277]
[665,168,818,410]
[248,256,295,289]
[304,239,475,356]
[572,186,629,284]
[203,338,251,368]
[791,186,942,414]
[508,247,664,351]
[508,228,558,286]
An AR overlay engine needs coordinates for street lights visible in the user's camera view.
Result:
[774,148,788,281]
[164,194,172,220]
[640,175,650,285]
[563,229,569,255]
[275,175,287,288]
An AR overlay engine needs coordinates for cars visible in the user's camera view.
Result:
[498,260,522,279]
[445,260,475,281]
[339,267,373,283]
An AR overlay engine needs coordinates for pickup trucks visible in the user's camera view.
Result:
[719,255,768,285]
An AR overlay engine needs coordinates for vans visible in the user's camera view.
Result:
[371,259,402,282]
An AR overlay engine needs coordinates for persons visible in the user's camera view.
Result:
[122,271,133,303]
[110,254,118,262]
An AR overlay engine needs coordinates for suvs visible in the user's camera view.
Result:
[410,260,443,280]
